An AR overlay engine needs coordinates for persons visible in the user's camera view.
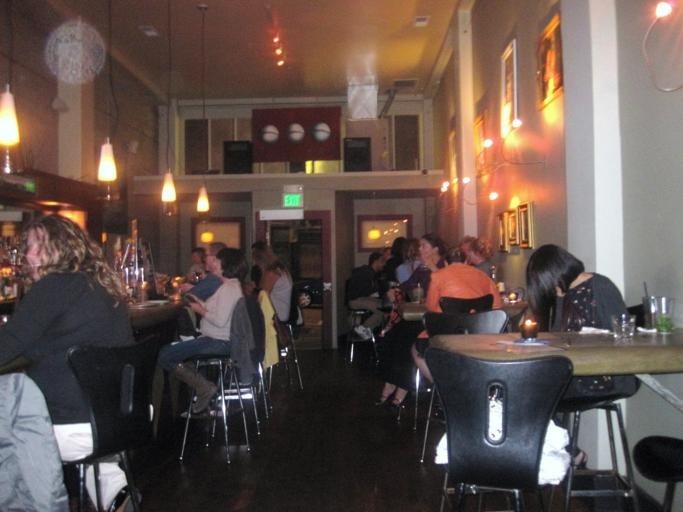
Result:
[412,247,507,390]
[250,237,293,326]
[0,214,141,512]
[179,242,228,300]
[186,247,207,279]
[159,247,249,416]
[460,237,496,281]
[524,242,638,475]
[384,236,412,268]
[345,252,385,342]
[371,233,446,413]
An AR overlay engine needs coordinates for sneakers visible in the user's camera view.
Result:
[354,324,373,340]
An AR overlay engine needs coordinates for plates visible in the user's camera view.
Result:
[150,299,172,308]
[135,298,158,309]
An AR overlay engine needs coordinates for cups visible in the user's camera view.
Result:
[625,316,636,345]
[651,296,673,337]
[610,312,626,341]
[411,283,423,310]
[644,295,660,333]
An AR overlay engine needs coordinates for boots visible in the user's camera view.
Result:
[175,360,218,414]
[190,417,222,460]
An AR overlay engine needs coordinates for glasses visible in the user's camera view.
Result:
[202,253,215,257]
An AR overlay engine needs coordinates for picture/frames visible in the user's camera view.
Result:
[500,37,518,129]
[518,201,534,249]
[508,211,520,246]
[497,211,510,254]
[532,12,565,110]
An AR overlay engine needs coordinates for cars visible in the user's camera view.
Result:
[289,277,323,308]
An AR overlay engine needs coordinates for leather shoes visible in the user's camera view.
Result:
[109,485,142,512]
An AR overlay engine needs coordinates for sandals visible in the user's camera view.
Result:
[574,451,588,468]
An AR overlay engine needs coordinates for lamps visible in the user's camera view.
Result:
[96,0,119,182]
[642,1,683,93]
[1,0,21,152]
[160,1,178,205]
[196,3,212,211]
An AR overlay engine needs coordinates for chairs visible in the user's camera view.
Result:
[342,280,379,367]
[560,299,645,512]
[267,282,305,393]
[409,290,496,434]
[179,295,260,466]
[65,331,170,512]
[419,308,508,464]
[422,347,574,512]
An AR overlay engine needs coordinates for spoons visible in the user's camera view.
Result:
[641,280,650,299]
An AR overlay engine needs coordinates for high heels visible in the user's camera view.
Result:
[387,390,412,412]
[373,392,397,407]
